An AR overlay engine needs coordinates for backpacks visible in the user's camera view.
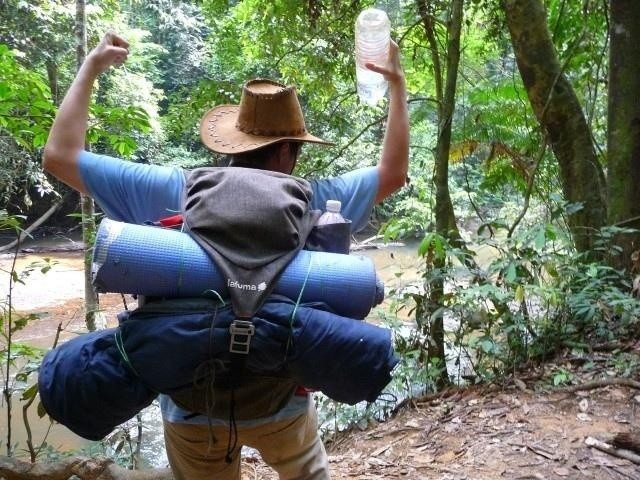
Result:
[162,164,324,426]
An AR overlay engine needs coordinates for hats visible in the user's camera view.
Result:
[197,75,339,158]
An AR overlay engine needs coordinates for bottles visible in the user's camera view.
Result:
[354,6,391,109]
[314,199,347,227]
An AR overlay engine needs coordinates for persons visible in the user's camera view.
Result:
[42,29,410,480]
